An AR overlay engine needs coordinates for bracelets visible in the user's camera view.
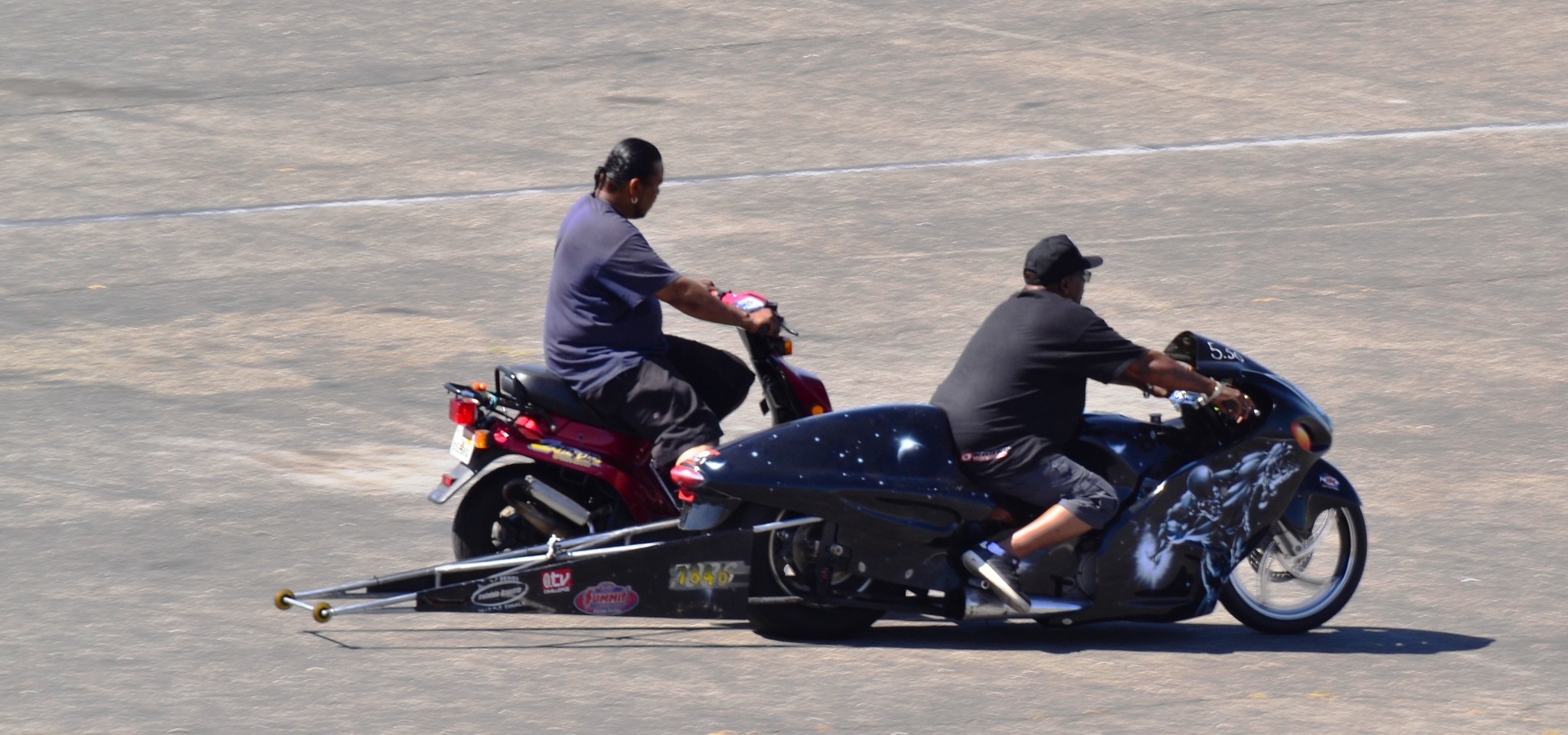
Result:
[1143,386,1154,397]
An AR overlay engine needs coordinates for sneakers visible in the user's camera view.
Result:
[962,540,1031,615]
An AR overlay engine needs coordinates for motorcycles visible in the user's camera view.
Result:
[660,330,1367,644]
[422,288,835,558]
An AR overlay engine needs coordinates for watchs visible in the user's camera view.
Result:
[1206,376,1226,403]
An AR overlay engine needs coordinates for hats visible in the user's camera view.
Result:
[1024,234,1103,285]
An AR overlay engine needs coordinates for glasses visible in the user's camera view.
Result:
[1069,269,1091,282]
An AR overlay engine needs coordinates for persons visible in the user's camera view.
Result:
[932,232,1255,618]
[543,136,780,492]
